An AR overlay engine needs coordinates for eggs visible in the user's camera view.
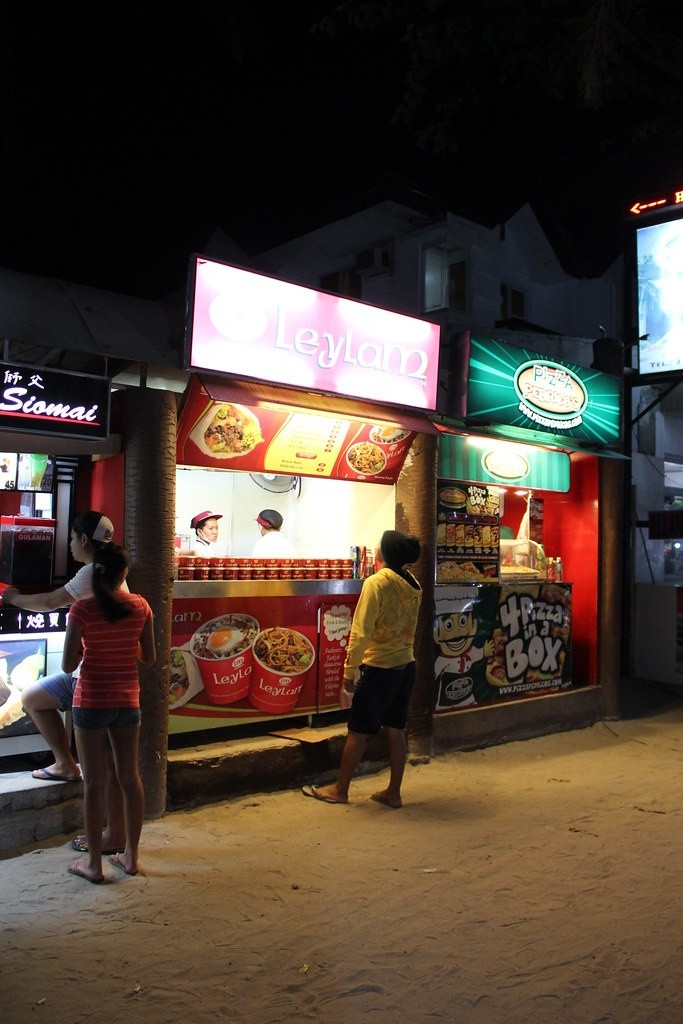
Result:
[205,625,245,654]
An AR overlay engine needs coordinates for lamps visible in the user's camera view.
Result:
[622,333,651,350]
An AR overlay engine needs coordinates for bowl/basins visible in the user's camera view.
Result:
[438,487,468,508]
[177,556,354,581]
[190,613,260,704]
[249,628,315,714]
[446,677,473,699]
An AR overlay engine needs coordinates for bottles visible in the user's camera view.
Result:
[546,557,563,583]
[537,544,546,579]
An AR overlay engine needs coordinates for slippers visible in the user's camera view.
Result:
[32,768,81,783]
[303,784,336,803]
[370,790,402,809]
[109,852,139,876]
[67,861,105,883]
[72,835,125,855]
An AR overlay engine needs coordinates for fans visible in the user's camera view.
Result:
[247,473,302,499]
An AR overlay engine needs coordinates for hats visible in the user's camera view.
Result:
[74,511,124,549]
[190,511,223,529]
[254,509,283,529]
[381,530,420,572]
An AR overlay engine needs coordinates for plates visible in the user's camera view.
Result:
[369,426,411,444]
[346,442,386,475]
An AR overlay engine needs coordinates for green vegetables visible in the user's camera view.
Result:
[169,650,185,703]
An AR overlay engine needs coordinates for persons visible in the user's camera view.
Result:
[252,509,297,557]
[2,511,132,854]
[302,530,422,808]
[61,542,156,884]
[191,511,227,557]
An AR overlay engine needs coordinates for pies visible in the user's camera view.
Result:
[189,403,264,458]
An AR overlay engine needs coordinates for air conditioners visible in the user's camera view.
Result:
[353,248,392,274]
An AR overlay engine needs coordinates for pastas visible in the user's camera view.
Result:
[256,627,312,674]
[350,444,384,474]
